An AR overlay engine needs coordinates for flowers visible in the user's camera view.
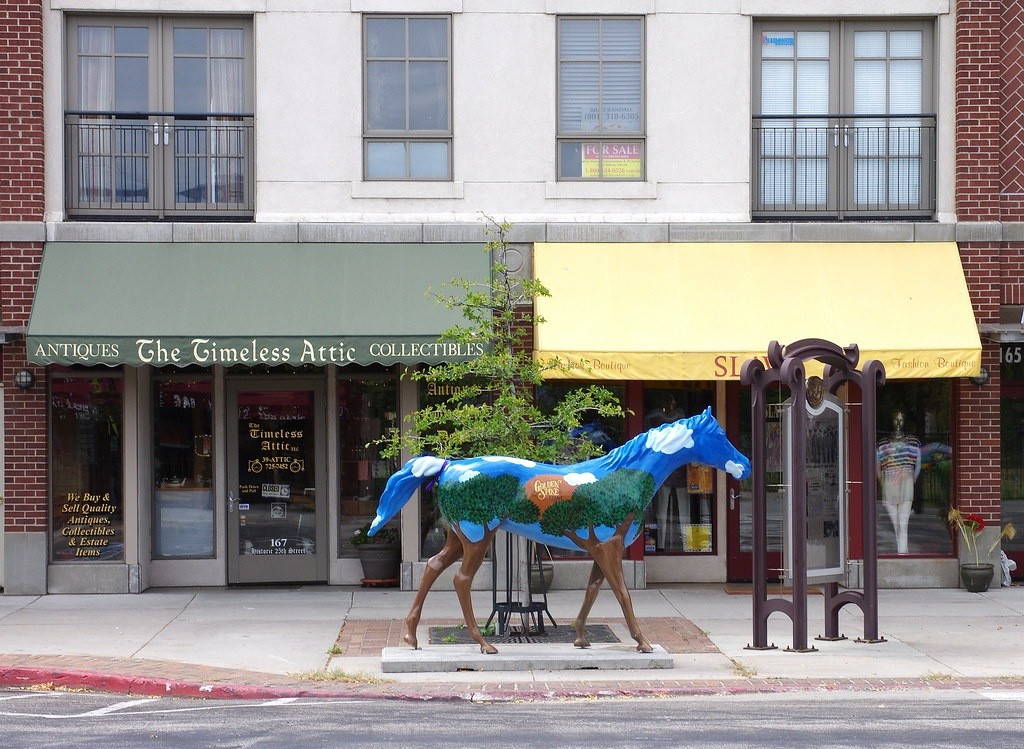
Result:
[948,504,1017,566]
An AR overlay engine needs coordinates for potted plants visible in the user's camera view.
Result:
[349,522,401,588]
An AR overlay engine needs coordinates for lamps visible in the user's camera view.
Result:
[969,366,990,385]
[14,369,33,389]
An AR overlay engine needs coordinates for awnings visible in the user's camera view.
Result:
[27,241,494,366]
[534,242,982,381]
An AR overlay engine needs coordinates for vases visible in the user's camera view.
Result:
[531,564,554,594]
[960,563,995,593]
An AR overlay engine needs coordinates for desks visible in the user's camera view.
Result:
[155,480,213,557]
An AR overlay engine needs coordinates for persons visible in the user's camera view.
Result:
[876,410,922,553]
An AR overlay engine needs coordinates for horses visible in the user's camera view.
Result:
[366,406,751,654]
